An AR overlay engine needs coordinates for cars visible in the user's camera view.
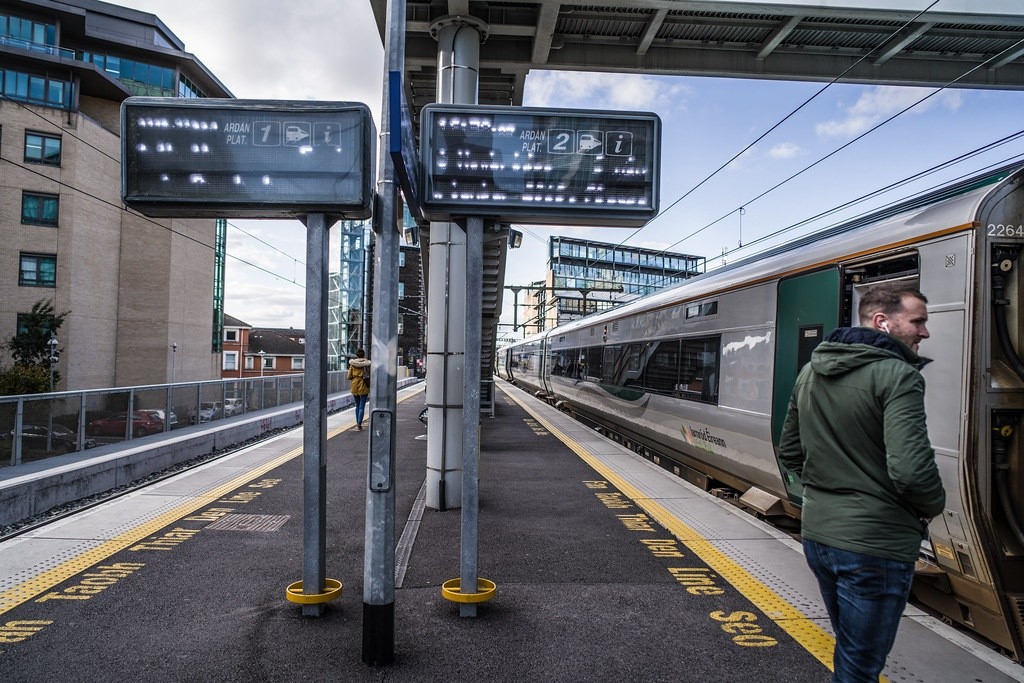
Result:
[10,422,96,451]
[225,398,248,418]
[87,410,164,438]
[138,409,179,430]
[189,401,222,425]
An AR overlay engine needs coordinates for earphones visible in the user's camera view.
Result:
[882,322,890,335]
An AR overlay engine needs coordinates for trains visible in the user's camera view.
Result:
[493,167,1024,667]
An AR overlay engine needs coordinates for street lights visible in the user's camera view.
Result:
[170,342,179,383]
[47,331,60,454]
[258,350,266,378]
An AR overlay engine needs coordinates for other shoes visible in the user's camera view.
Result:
[356,425,364,431]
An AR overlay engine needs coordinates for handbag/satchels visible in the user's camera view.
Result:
[362,365,371,388]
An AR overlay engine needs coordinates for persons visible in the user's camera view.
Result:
[524,359,581,377]
[778,280,944,683]
[347,349,371,430]
